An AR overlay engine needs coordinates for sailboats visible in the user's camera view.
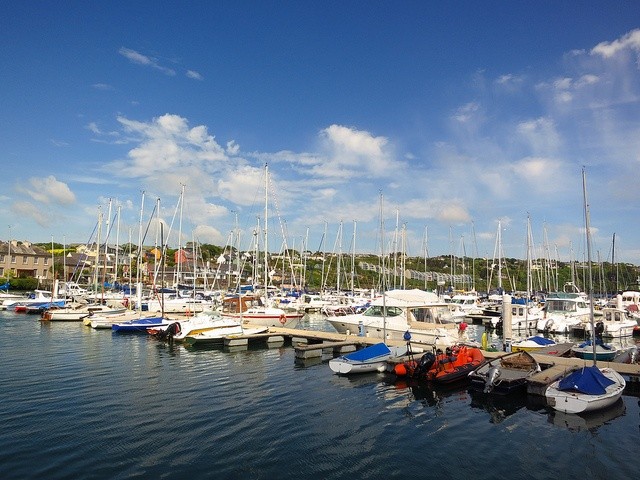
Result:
[511,335,573,356]
[330,194,481,315]
[547,164,627,415]
[31,299,71,311]
[469,211,640,336]
[173,317,268,346]
[330,299,467,347]
[572,337,638,363]
[233,306,302,326]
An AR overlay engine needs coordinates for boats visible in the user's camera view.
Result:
[86,313,156,329]
[394,342,482,384]
[45,305,113,323]
[468,351,540,395]
[326,342,413,377]
[0,295,53,313]
[110,318,172,332]
